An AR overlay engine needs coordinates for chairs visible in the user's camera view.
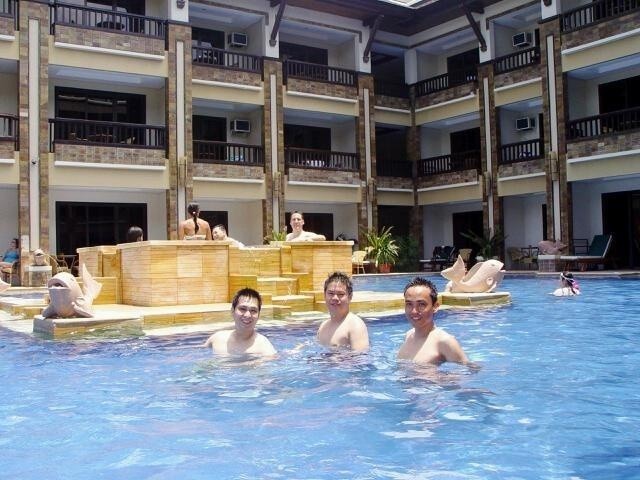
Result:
[0,260,19,286]
[352,250,367,275]
[560,235,613,272]
[418,246,456,272]
[518,247,539,272]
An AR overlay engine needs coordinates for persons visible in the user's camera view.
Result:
[212,224,246,248]
[200,286,278,356]
[316,270,370,351]
[396,277,469,365]
[553,271,581,296]
[126,225,143,242]
[0,238,20,280]
[286,209,326,242]
[177,202,211,240]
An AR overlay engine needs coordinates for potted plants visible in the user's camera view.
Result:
[459,230,510,263]
[363,225,399,273]
